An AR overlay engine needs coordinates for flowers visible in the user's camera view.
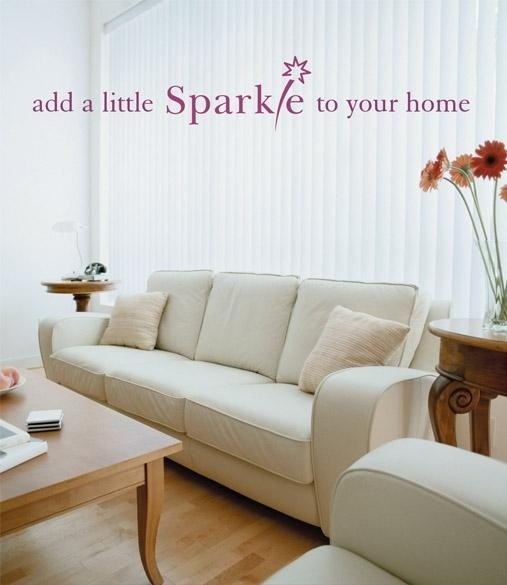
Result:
[420,140,507,325]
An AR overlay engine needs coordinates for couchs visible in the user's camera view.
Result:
[37,266,486,544]
[260,435,507,585]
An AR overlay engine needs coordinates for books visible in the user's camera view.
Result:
[25,410,63,433]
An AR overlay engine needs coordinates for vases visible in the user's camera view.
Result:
[483,272,507,335]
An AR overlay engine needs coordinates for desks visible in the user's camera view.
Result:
[427,318,507,457]
[40,279,118,312]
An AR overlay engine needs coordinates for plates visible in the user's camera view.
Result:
[0,372,26,396]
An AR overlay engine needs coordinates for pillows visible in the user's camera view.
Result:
[98,289,168,350]
[299,303,408,398]
